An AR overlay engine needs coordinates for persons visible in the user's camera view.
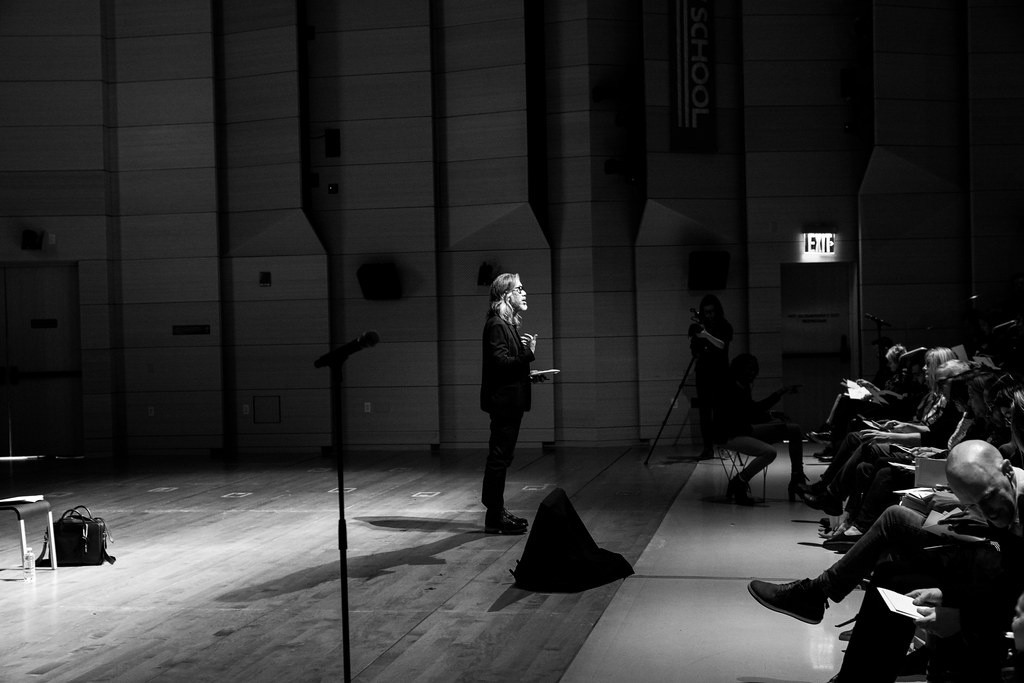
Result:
[692,296,1023,682]
[481,273,550,534]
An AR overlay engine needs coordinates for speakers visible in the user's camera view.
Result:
[19,229,49,253]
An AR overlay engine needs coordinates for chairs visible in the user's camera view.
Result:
[0,456,58,571]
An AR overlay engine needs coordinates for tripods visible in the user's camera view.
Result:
[643,355,729,460]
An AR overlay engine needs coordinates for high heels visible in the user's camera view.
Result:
[787,472,809,502]
[725,473,755,506]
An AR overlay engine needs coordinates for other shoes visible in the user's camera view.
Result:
[897,645,933,677]
[838,628,854,641]
[818,525,847,539]
[696,446,714,461]
[720,449,733,460]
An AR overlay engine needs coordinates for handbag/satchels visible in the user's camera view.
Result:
[34,503,116,567]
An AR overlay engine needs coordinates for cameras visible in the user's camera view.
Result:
[687,309,709,359]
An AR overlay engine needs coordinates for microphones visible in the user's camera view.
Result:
[313,329,381,369]
[864,312,892,328]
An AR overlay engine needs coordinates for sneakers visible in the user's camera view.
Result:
[826,672,839,683]
[792,481,827,502]
[802,488,844,516]
[499,509,528,528]
[805,430,833,445]
[748,578,829,625]
[822,532,865,554]
[814,448,836,462]
[483,508,527,534]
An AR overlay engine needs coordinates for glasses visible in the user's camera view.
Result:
[1002,415,1013,424]
[983,401,996,412]
[936,377,950,387]
[512,286,524,295]
